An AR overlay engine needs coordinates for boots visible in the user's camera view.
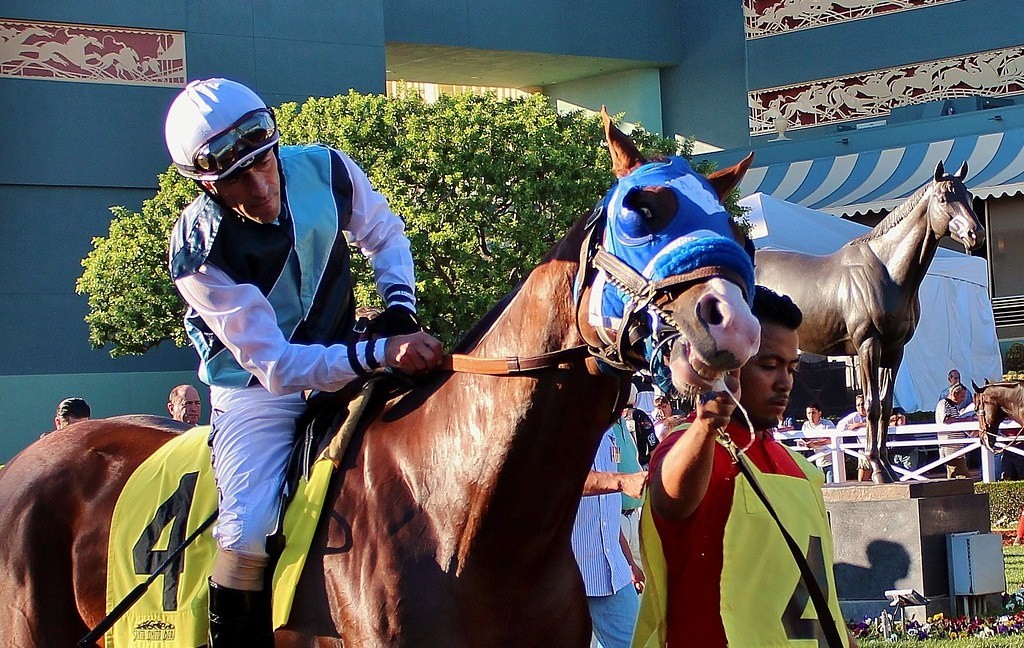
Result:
[206,576,269,648]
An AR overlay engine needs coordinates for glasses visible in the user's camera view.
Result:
[171,102,277,176]
[855,403,864,406]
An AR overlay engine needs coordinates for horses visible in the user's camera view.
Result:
[966,376,1024,446]
[0,106,764,648]
[754,159,985,487]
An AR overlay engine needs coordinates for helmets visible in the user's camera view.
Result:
[164,78,280,181]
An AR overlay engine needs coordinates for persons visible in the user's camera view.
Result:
[167,384,203,427]
[572,370,974,648]
[632,287,856,646]
[39,397,89,433]
[165,76,442,648]
